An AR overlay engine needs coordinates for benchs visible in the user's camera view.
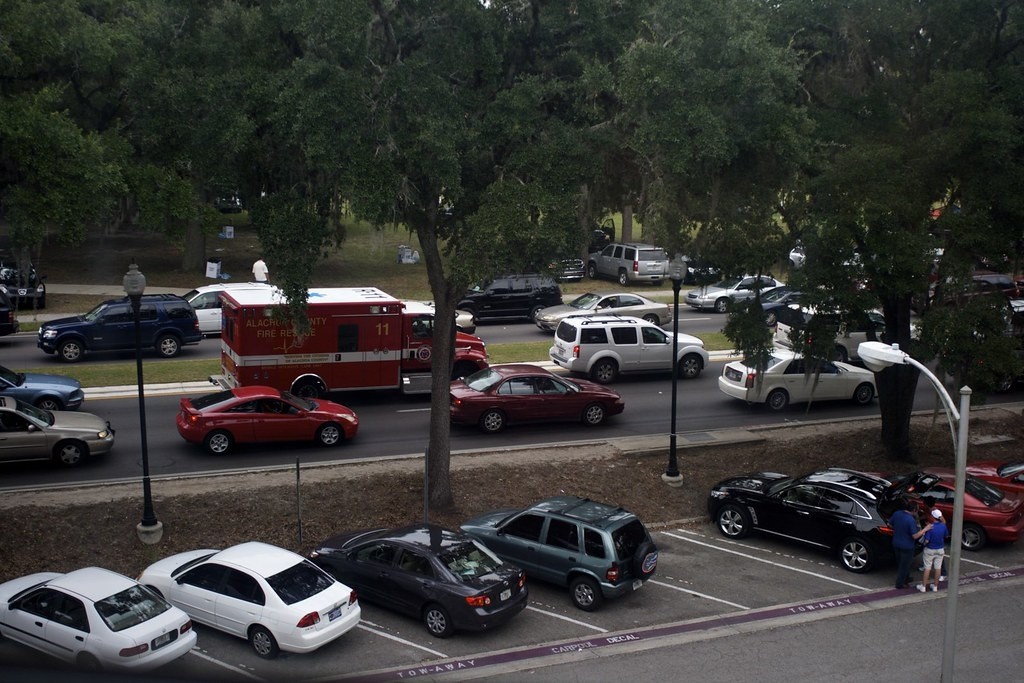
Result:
[108,600,155,624]
[449,550,481,573]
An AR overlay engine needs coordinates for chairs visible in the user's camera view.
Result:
[403,554,420,572]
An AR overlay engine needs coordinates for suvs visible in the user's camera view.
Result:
[455,278,564,322]
[548,315,711,379]
[538,215,670,286]
[705,464,940,574]
[183,282,276,335]
[38,293,203,358]
[459,494,659,612]
[773,305,919,363]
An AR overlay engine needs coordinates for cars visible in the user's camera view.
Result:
[0,365,115,471]
[719,349,877,410]
[137,540,363,658]
[680,245,1024,338]
[310,523,528,639]
[1,566,198,682]
[176,388,359,457]
[399,298,475,335]
[867,456,1024,551]
[448,362,625,429]
[0,258,46,337]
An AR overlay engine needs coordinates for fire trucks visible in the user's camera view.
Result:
[209,287,489,401]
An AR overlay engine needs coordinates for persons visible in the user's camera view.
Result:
[251,256,270,284]
[915,509,947,593]
[888,501,933,589]
[919,496,948,582]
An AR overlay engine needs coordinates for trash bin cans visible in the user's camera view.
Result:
[222,225,234,239]
[398,247,411,262]
[206,259,221,279]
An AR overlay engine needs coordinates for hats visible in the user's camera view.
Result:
[931,509,943,520]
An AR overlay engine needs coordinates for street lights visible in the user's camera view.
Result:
[666,253,686,476]
[858,338,971,683]
[122,263,160,526]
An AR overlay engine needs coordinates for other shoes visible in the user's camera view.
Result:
[905,577,913,583]
[916,584,926,593]
[930,584,937,592]
[939,576,946,582]
[896,584,905,589]
[919,567,925,571]
